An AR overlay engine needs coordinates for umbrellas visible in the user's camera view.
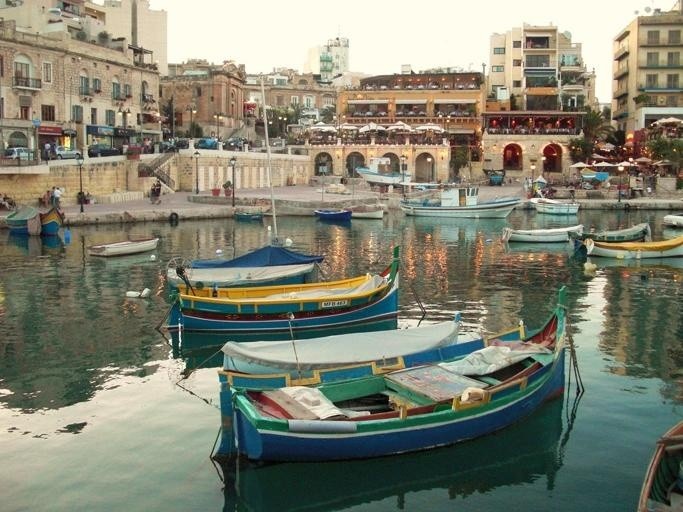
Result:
[567,154,674,168]
[649,117,683,129]
[305,121,337,134]
[335,123,360,130]
[387,120,414,131]
[415,122,448,132]
[357,122,386,133]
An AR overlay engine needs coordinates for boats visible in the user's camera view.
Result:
[4,204,63,235]
[344,206,384,219]
[530,198,580,214]
[86,234,158,256]
[500,222,583,243]
[154,308,397,377]
[313,209,351,222]
[577,233,682,258]
[583,256,683,280]
[567,222,649,240]
[400,186,520,219]
[157,243,397,323]
[195,285,568,458]
[662,210,683,228]
[6,232,63,258]
[210,312,458,375]
[221,394,567,511]
[637,413,682,512]
[354,167,412,186]
[161,244,325,289]
[232,210,263,222]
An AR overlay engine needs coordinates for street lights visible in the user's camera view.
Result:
[184,104,197,138]
[212,111,223,142]
[399,152,407,193]
[332,112,345,138]
[617,165,624,202]
[76,156,85,213]
[277,114,287,139]
[193,151,199,195]
[530,162,536,195]
[229,155,236,207]
[426,156,432,184]
[115,107,132,145]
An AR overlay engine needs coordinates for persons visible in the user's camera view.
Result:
[2,194,15,209]
[154,180,161,205]
[637,167,660,188]
[0,194,12,210]
[49,186,55,206]
[53,187,66,208]
[40,191,51,209]
[141,134,250,155]
[43,142,50,160]
[522,172,577,193]
[149,182,155,204]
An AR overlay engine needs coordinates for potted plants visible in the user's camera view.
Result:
[211,177,232,196]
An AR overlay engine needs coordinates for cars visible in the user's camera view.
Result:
[87,144,120,157]
[194,138,216,149]
[222,136,247,151]
[43,144,80,160]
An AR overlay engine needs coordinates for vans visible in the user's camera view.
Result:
[1,145,33,161]
